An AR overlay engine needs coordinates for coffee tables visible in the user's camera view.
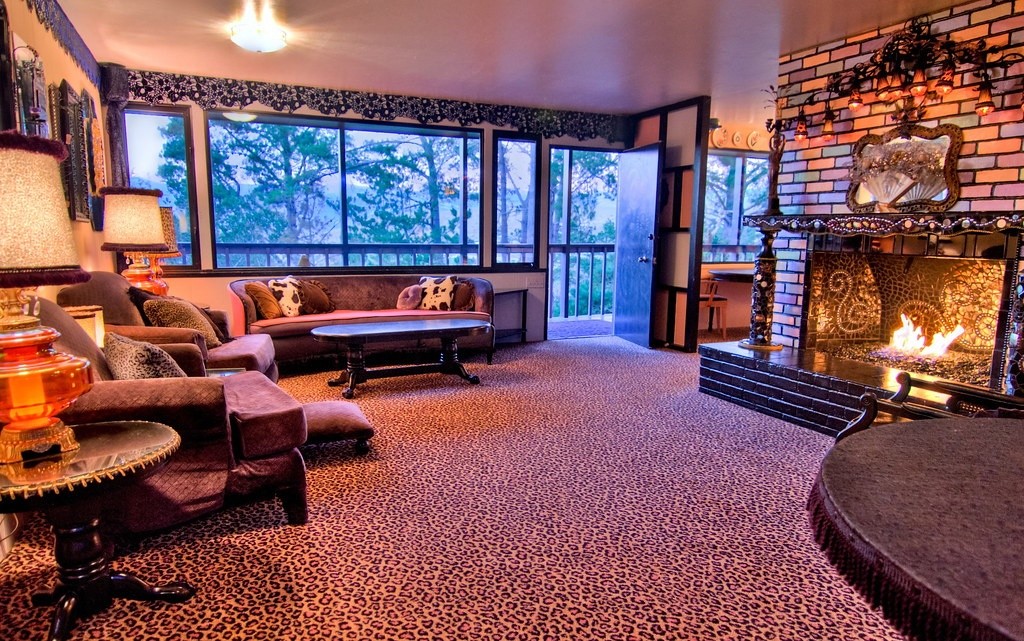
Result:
[311,319,495,398]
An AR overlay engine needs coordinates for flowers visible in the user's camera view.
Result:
[833,146,945,185]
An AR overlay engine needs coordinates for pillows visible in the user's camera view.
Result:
[126,285,222,349]
[103,330,188,380]
[244,274,336,320]
[396,274,476,311]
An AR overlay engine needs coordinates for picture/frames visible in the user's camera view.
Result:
[58,77,90,223]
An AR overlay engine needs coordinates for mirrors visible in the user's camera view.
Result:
[846,118,964,212]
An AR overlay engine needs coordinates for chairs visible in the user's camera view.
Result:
[700,277,729,342]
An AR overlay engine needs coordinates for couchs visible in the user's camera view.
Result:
[19,294,308,560]
[57,267,279,384]
[227,274,496,365]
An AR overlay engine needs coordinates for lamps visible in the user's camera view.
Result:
[222,0,292,54]
[98,185,169,296]
[121,206,182,297]
[794,13,1024,143]
[0,128,92,465]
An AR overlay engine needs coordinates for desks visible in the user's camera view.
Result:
[709,270,755,283]
[0,420,199,641]
[809,417,1024,641]
